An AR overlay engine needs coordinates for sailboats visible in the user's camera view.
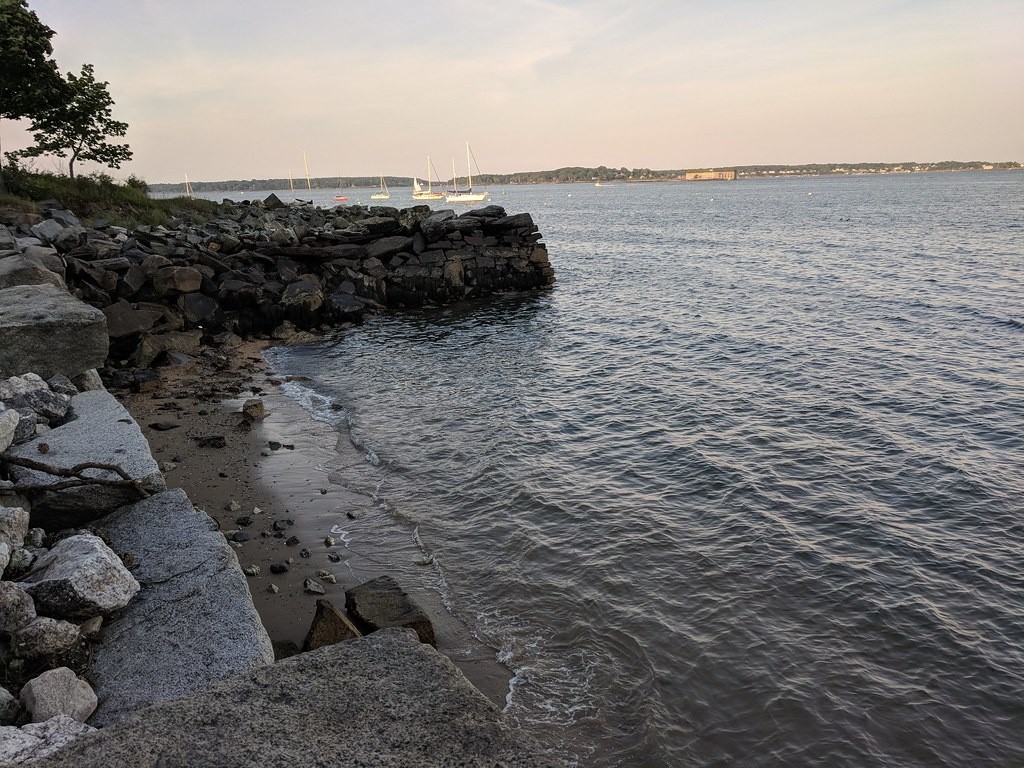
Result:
[446,141,488,202]
[369,172,390,199]
[288,150,314,208]
[413,154,445,200]
